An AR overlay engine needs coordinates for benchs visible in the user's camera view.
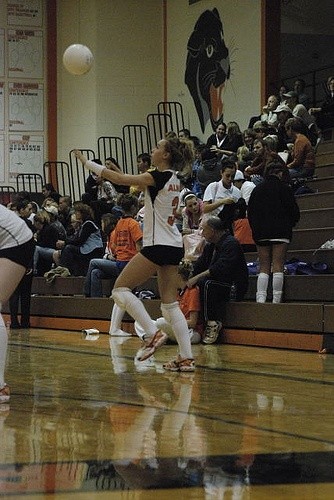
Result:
[0,139,334,353]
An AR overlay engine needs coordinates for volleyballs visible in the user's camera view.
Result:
[62,42,94,75]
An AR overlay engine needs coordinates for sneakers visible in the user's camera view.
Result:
[0,384,11,404]
[137,329,168,362]
[164,372,197,385]
[202,320,222,344]
[162,354,196,372]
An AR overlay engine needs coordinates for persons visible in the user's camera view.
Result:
[72,136,198,372]
[5,76,323,345]
[0,204,35,403]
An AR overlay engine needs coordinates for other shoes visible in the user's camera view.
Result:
[108,327,132,337]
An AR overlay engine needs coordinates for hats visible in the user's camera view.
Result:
[41,206,58,215]
[282,91,295,98]
[272,105,292,113]
